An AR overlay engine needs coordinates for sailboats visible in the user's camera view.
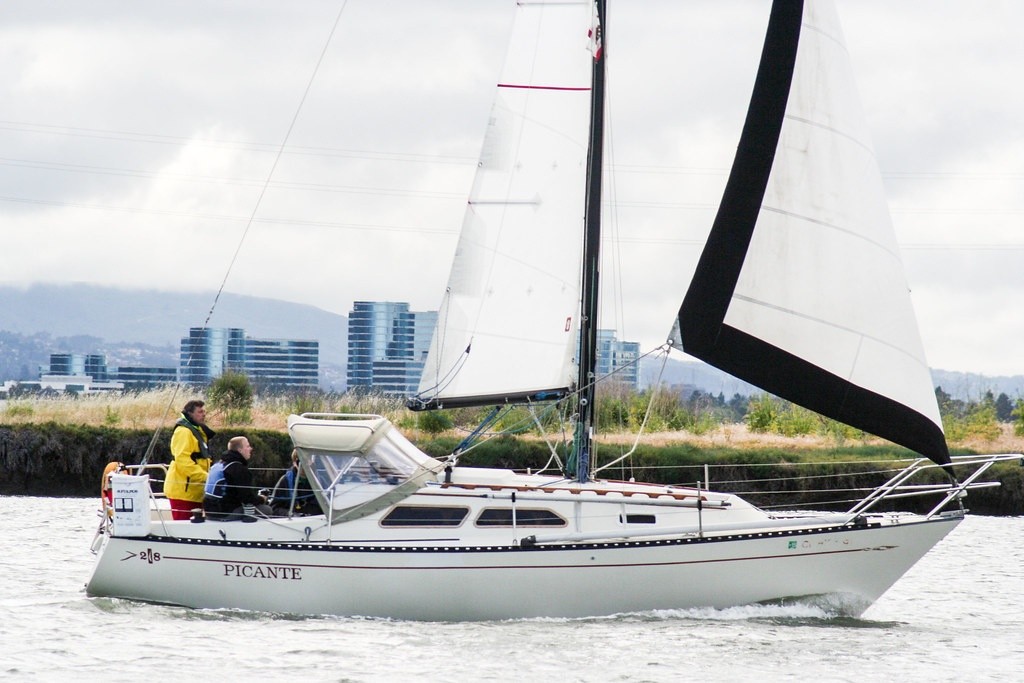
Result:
[81,1,1024,620]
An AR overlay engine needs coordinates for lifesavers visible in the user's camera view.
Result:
[101,461,128,516]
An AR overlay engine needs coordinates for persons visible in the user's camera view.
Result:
[270,448,330,517]
[202,436,273,521]
[162,399,217,520]
